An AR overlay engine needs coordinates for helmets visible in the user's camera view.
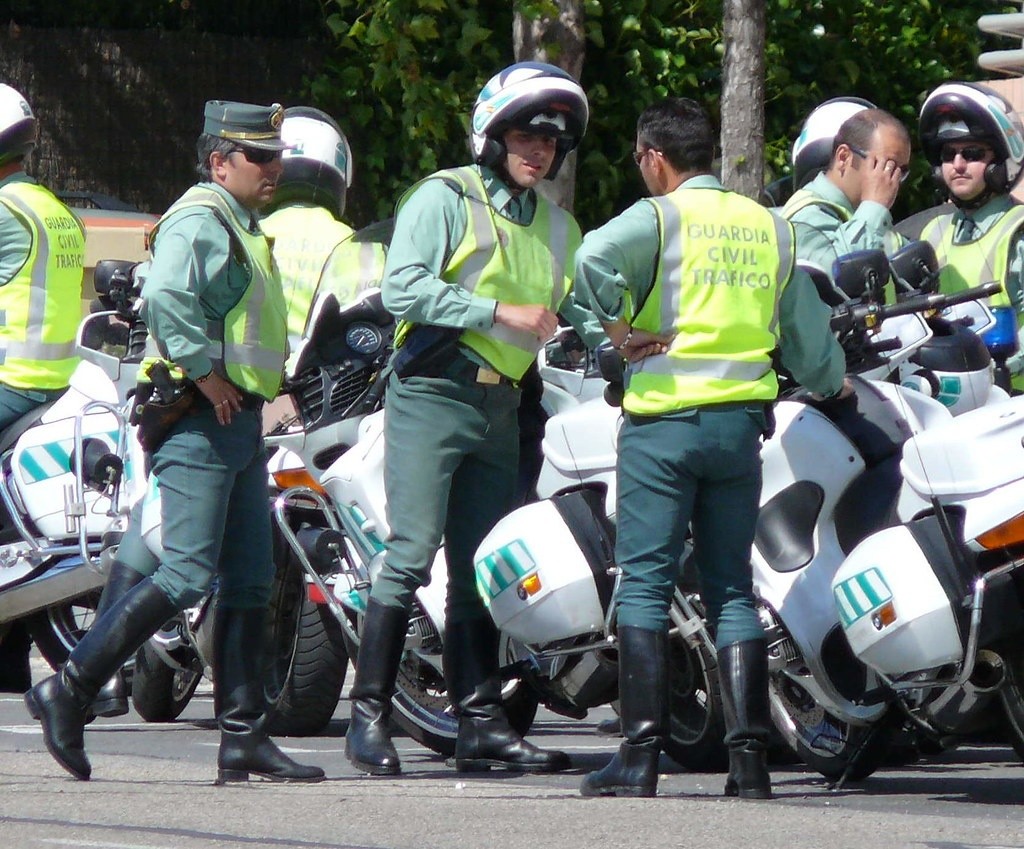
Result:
[468,60,589,183]
[259,105,352,219]
[0,82,35,165]
[790,95,880,191]
[917,79,1024,194]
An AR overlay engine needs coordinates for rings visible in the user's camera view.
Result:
[215,403,221,408]
[222,399,227,405]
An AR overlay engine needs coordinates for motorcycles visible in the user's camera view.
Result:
[795,252,955,431]
[261,219,968,782]
[1,259,350,732]
[887,240,1013,415]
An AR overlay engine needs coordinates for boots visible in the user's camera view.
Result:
[344,597,408,776]
[24,576,178,782]
[580,626,668,797]
[211,604,327,783]
[716,637,771,799]
[82,558,146,725]
[442,615,573,774]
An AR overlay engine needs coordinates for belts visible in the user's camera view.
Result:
[402,361,513,388]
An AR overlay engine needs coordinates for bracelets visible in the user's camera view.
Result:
[617,323,633,351]
[196,370,215,383]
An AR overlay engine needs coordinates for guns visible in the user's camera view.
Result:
[136,358,193,454]
[362,330,394,414]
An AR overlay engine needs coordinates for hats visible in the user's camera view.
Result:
[203,100,294,152]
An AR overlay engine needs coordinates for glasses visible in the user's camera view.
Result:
[230,147,283,164]
[630,149,664,166]
[835,144,911,185]
[934,145,995,162]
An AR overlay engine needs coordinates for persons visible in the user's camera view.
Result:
[77,106,389,725]
[0,84,86,694]
[572,97,854,798]
[757,81,1024,399]
[345,61,615,775]
[25,100,325,783]
